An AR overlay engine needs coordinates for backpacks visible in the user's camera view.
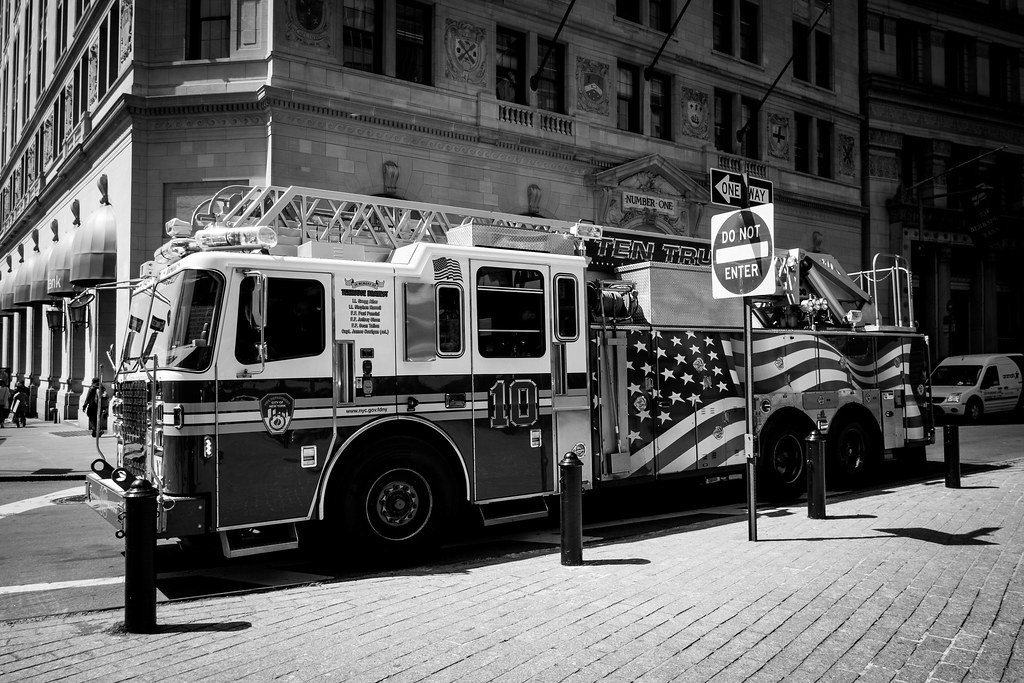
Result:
[95,388,109,407]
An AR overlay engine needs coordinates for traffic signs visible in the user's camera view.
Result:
[708,167,775,209]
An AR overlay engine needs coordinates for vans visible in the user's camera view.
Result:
[926,353,1024,425]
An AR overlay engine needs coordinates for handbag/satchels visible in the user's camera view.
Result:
[8,412,14,423]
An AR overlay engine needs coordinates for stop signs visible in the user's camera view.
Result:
[710,203,776,300]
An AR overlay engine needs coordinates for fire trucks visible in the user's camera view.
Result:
[68,183,932,578]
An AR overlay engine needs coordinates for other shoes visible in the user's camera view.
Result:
[16,422,20,428]
[23,422,26,427]
[92,428,104,437]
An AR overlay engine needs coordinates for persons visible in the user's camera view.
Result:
[83,377,107,438]
[1,375,29,429]
